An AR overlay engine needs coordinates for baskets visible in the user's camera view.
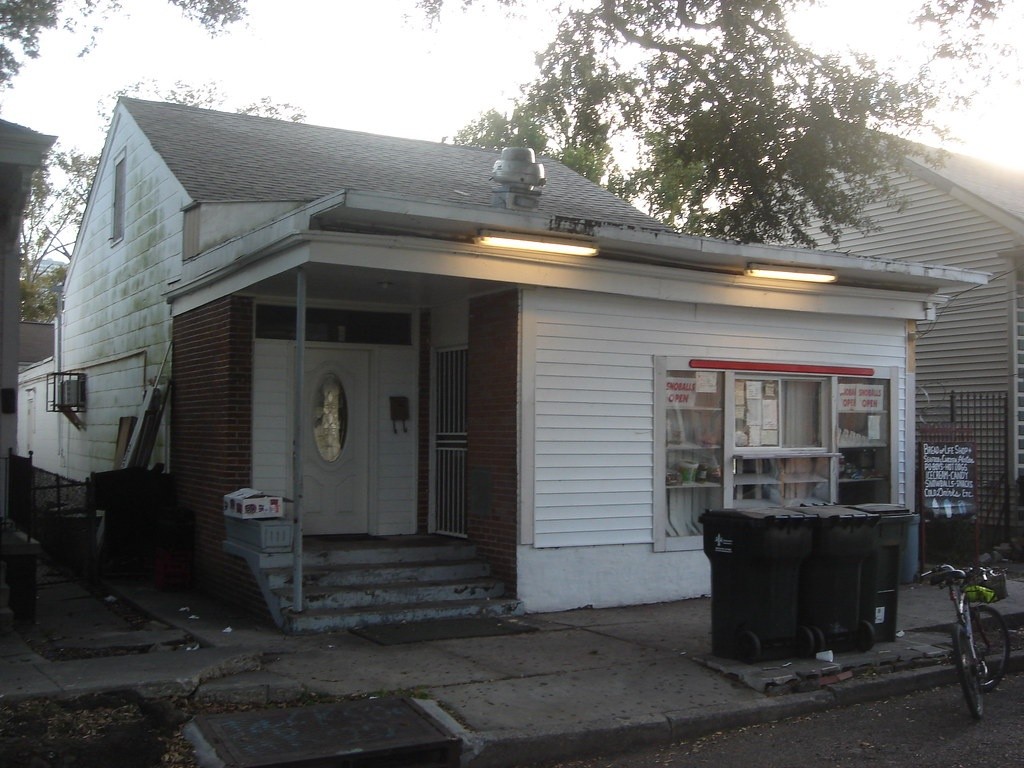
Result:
[959,568,1008,603]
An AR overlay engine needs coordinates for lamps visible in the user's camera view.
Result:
[744,262,838,283]
[471,230,600,258]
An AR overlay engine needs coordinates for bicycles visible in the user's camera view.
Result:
[919,563,1009,719]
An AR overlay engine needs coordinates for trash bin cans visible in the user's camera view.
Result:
[696,502,917,662]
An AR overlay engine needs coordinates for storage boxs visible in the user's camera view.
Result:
[223,488,296,569]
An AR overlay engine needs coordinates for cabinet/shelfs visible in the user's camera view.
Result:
[666,371,891,536]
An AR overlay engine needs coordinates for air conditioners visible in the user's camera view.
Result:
[58,380,85,407]
